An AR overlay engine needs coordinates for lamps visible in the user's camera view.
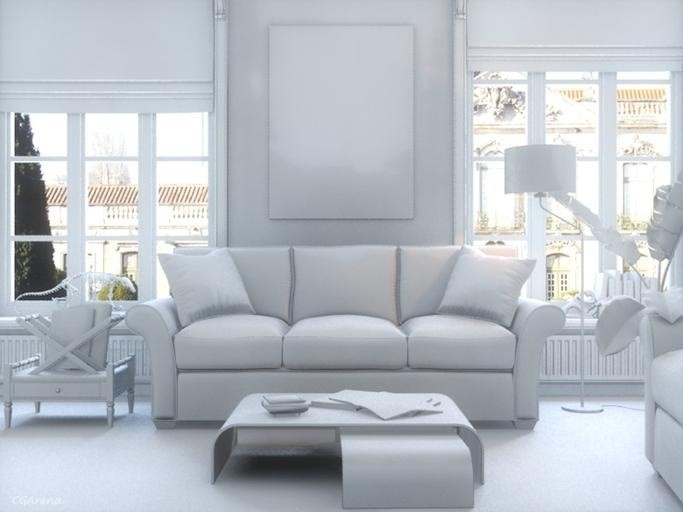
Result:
[502,143,605,413]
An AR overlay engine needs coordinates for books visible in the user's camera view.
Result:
[308,388,444,421]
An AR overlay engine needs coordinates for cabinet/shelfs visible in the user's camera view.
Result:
[2,352,138,430]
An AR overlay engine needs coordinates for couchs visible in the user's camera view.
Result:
[123,241,566,428]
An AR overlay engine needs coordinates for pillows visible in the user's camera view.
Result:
[152,247,255,327]
[434,243,536,329]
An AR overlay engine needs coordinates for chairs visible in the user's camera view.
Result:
[641,279,683,501]
